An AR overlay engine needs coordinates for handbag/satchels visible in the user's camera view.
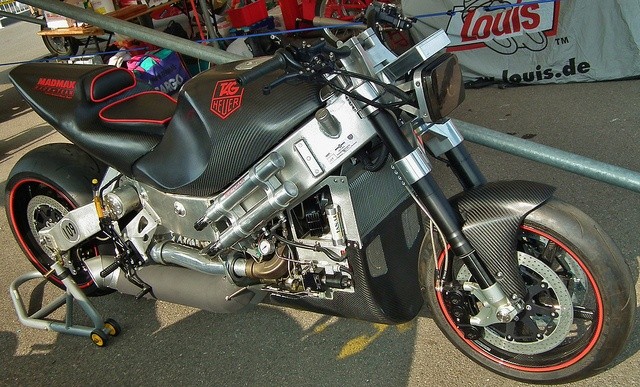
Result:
[128,48,190,94]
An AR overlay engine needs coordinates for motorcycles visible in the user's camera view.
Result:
[4,0,636,384]
